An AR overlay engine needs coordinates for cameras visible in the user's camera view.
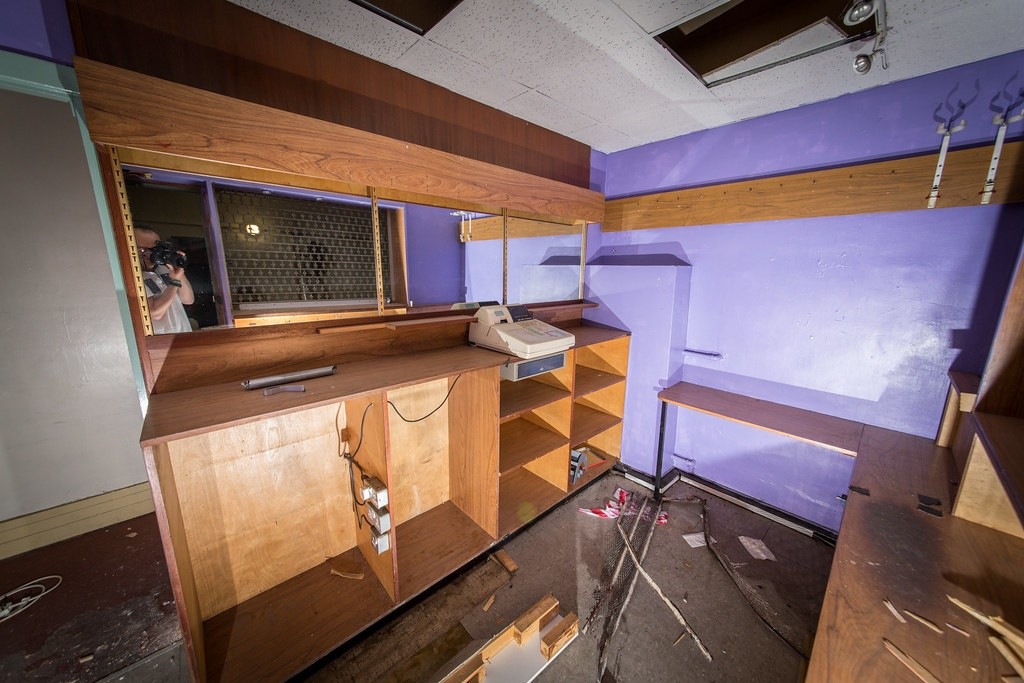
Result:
[150,240,186,268]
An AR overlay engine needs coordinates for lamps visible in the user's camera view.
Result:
[851,47,885,75]
[840,0,879,26]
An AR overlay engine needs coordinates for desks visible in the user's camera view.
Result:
[651,380,865,506]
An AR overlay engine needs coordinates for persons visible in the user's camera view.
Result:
[134,225,194,335]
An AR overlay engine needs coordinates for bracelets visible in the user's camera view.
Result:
[168,279,182,288]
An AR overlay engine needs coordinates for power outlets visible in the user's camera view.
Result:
[363,476,390,555]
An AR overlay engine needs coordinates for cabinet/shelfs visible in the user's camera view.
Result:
[139,332,630,683]
[803,240,1024,683]
